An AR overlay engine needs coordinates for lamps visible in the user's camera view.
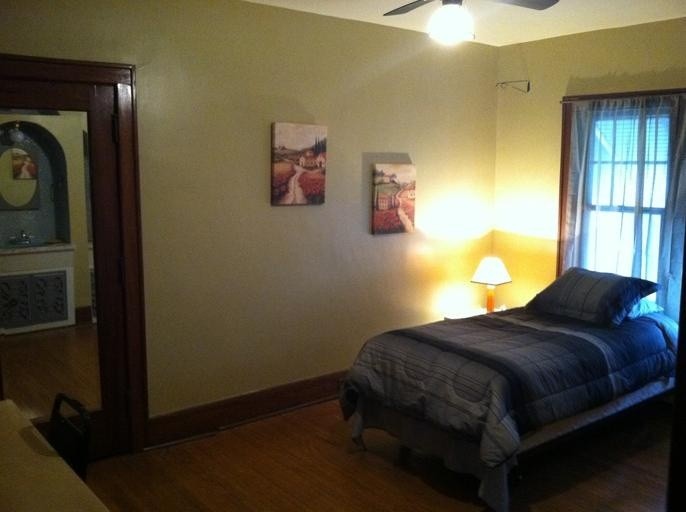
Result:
[470,256,511,313]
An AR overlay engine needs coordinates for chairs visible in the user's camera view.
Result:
[35,394,88,481]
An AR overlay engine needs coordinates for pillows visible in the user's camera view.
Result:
[628,298,664,320]
[525,267,662,327]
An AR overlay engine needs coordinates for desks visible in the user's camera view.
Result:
[0,398,111,512]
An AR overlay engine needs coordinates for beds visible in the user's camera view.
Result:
[338,306,679,512]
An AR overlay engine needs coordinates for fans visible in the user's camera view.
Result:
[383,0,560,16]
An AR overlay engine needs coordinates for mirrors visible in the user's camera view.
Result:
[0,141,40,210]
